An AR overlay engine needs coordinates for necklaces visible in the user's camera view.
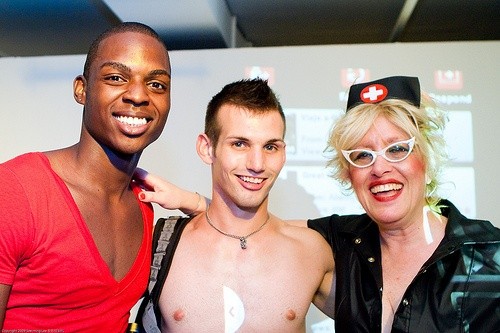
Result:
[205,202,271,250]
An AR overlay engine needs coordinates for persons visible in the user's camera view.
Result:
[132,76,500,333]
[152,78,336,333]
[0,21,172,333]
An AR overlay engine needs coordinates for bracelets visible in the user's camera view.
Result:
[184,192,202,217]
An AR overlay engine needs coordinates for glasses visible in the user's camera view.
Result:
[341,134,417,168]
